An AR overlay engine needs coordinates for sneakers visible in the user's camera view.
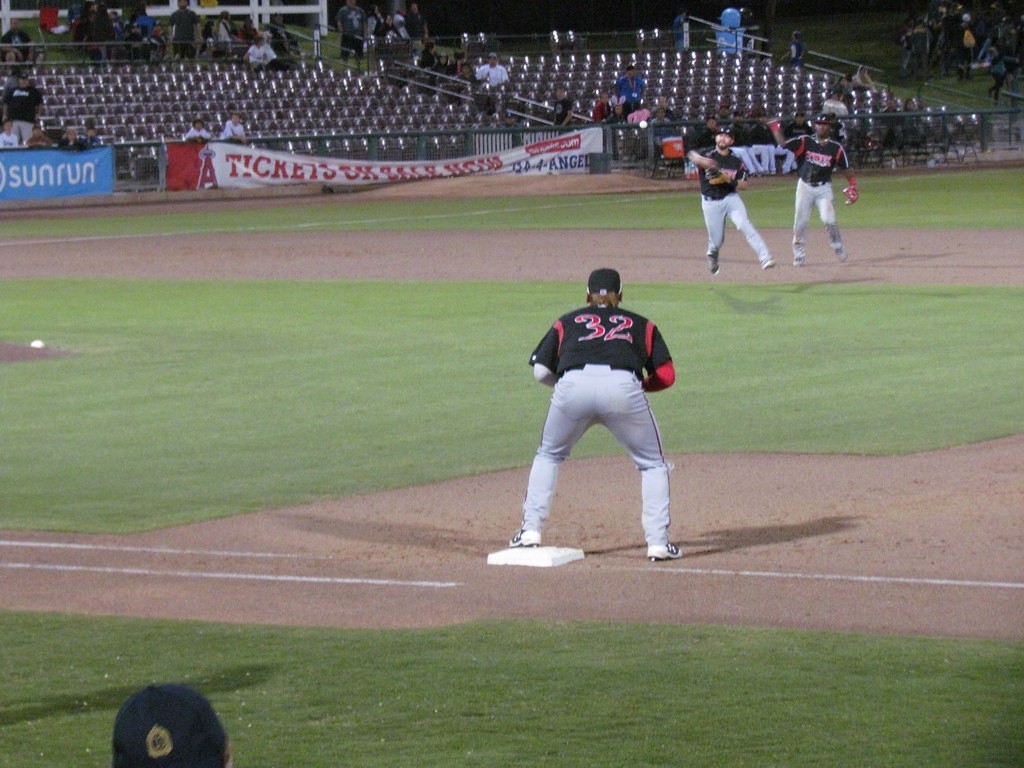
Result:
[707,251,720,276]
[647,542,682,559]
[759,257,775,270]
[509,530,541,547]
[834,247,848,263]
[793,257,806,266]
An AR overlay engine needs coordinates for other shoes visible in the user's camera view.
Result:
[750,165,797,178]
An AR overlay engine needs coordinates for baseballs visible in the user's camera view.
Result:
[30,339,45,348]
[640,120,648,128]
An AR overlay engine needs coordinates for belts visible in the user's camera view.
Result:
[805,180,826,188]
[559,365,641,382]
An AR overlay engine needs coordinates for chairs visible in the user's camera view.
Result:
[0,50,980,179]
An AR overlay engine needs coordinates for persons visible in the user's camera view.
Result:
[420,42,510,93]
[185,118,211,142]
[72,0,300,70]
[767,113,858,267]
[593,65,675,125]
[689,64,924,176]
[672,8,686,49]
[509,268,683,560]
[790,30,805,66]
[337,0,428,63]
[220,112,246,144]
[0,16,99,150]
[696,126,775,277]
[553,85,573,127]
[938,0,1024,105]
[112,684,233,768]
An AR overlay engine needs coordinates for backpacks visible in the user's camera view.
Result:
[964,29,975,48]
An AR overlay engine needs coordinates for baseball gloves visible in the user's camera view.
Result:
[842,185,859,205]
[704,166,731,185]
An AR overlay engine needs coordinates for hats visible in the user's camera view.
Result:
[706,104,806,120]
[488,52,498,59]
[714,126,733,138]
[586,269,622,295]
[112,684,226,768]
[812,114,829,123]
